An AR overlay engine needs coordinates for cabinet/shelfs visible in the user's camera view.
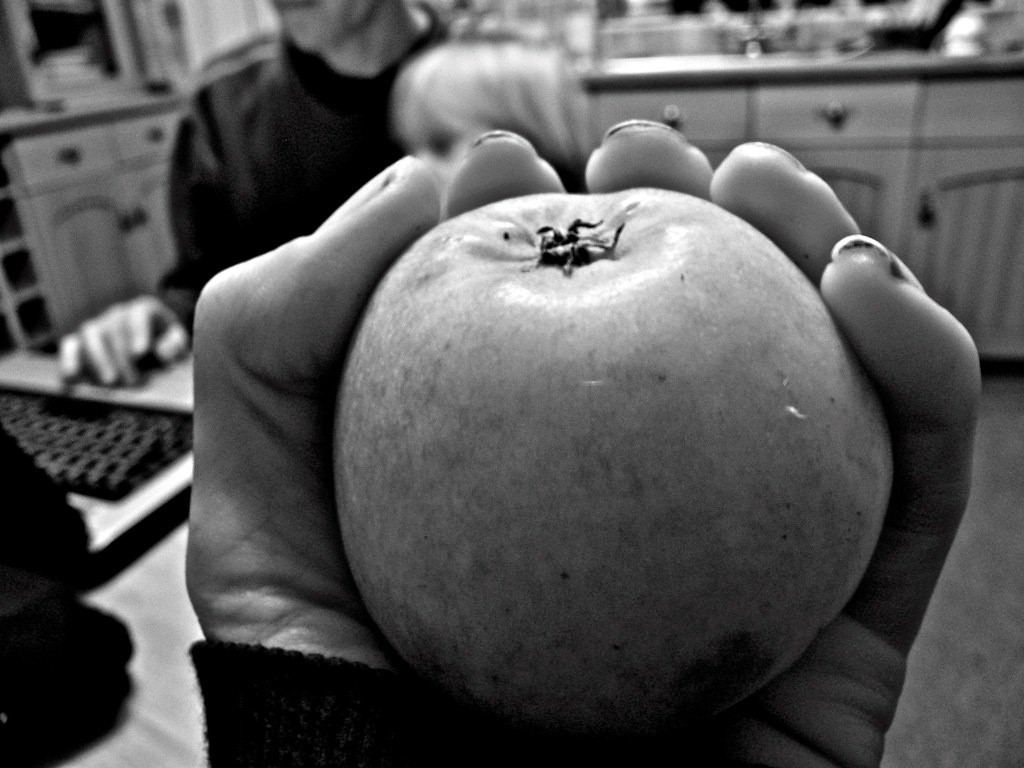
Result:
[576,55,1024,356]
[0,90,192,358]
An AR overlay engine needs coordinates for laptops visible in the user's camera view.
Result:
[0,350,195,564]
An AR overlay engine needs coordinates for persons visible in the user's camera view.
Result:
[0,425,135,768]
[185,119,984,768]
[395,39,597,195]
[58,0,448,388]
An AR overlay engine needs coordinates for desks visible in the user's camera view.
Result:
[0,353,212,768]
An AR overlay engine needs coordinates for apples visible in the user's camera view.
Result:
[334,186,894,739]
